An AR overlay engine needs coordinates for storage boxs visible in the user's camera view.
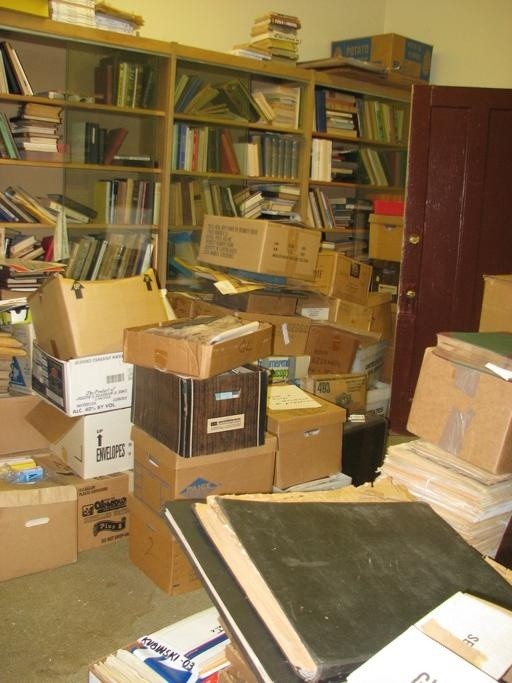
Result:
[404,343,512,474]
[0,210,404,596]
[475,269,512,333]
[330,29,436,81]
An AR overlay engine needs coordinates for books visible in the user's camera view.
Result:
[372,439,512,562]
[83,600,236,683]
[0,178,163,291]
[226,13,302,67]
[306,187,374,230]
[314,89,405,143]
[310,138,388,186]
[50,0,146,36]
[173,124,299,180]
[0,41,156,168]
[174,74,301,129]
[170,180,301,226]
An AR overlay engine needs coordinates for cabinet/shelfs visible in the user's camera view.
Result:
[302,62,413,295]
[162,40,312,297]
[0,12,173,396]
